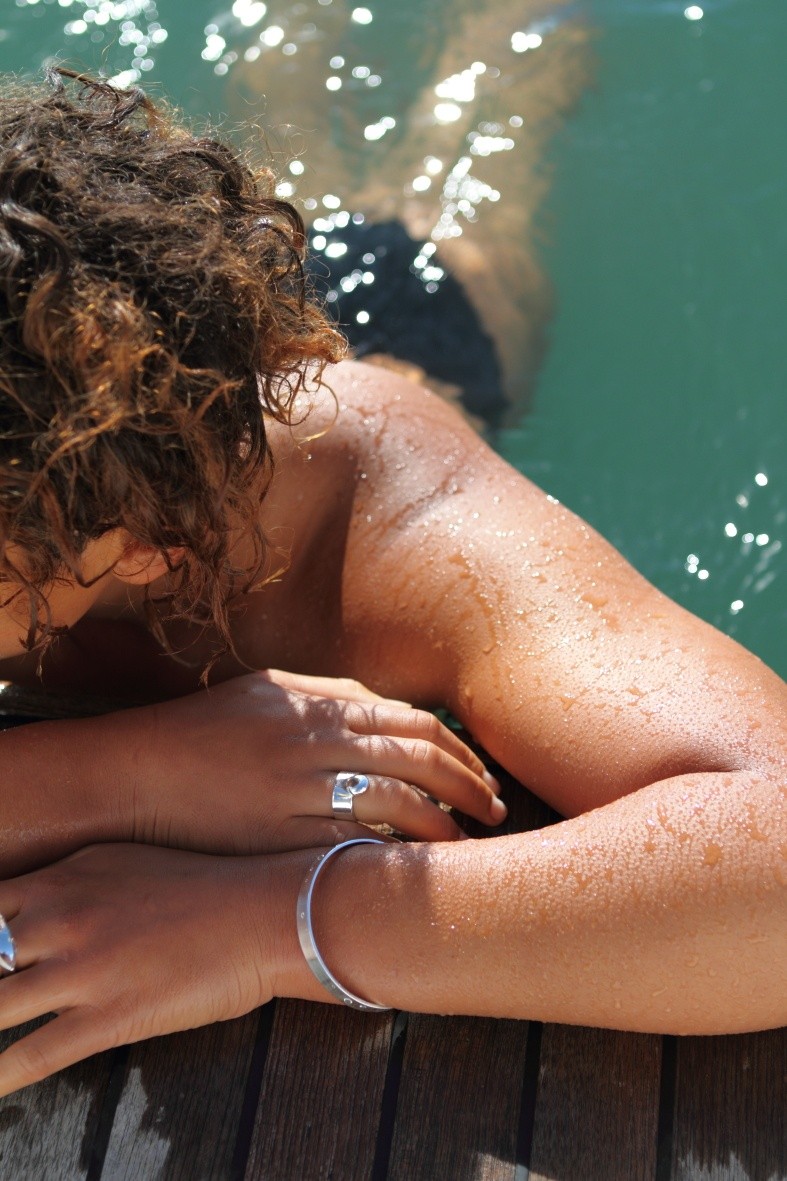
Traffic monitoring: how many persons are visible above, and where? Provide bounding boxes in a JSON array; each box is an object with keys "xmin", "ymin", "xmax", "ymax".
[{"xmin": 0, "ymin": 70, "xmax": 787, "ymax": 1102}]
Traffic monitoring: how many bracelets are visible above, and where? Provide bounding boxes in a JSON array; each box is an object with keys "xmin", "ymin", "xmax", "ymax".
[{"xmin": 297, "ymin": 840, "xmax": 392, "ymax": 1012}]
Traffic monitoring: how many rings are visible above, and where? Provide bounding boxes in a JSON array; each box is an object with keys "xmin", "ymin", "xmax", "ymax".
[
  {"xmin": 0, "ymin": 915, "xmax": 14, "ymax": 975},
  {"xmin": 332, "ymin": 771, "xmax": 368, "ymax": 820}
]
